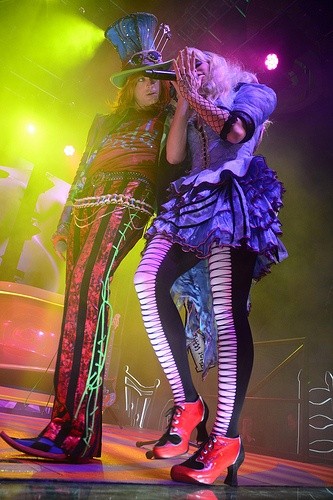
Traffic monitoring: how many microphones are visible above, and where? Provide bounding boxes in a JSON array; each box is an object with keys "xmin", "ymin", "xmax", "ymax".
[{"xmin": 142, "ymin": 69, "xmax": 176, "ymax": 81}]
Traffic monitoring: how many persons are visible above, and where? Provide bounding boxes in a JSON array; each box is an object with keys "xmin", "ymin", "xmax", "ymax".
[
  {"xmin": 0, "ymin": 12, "xmax": 175, "ymax": 463},
  {"xmin": 132, "ymin": 45, "xmax": 289, "ymax": 489}
]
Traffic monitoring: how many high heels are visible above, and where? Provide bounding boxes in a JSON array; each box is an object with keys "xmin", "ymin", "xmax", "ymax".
[
  {"xmin": 152, "ymin": 393, "xmax": 209, "ymax": 459},
  {"xmin": 170, "ymin": 435, "xmax": 245, "ymax": 487}
]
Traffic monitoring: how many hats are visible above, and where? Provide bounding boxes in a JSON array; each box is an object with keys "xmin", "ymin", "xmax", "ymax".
[{"xmin": 104, "ymin": 12, "xmax": 175, "ymax": 89}]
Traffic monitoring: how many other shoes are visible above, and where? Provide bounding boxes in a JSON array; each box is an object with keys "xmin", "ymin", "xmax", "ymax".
[{"xmin": 0, "ymin": 431, "xmax": 93, "ymax": 463}]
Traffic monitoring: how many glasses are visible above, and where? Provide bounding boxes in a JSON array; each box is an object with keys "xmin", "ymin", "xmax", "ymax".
[
  {"xmin": 195, "ymin": 61, "xmax": 202, "ymax": 68},
  {"xmin": 121, "ymin": 50, "xmax": 162, "ymax": 70}
]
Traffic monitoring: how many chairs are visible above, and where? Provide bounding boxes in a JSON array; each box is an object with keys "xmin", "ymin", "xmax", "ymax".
[{"xmin": 121, "ymin": 365, "xmax": 161, "ymax": 428}]
